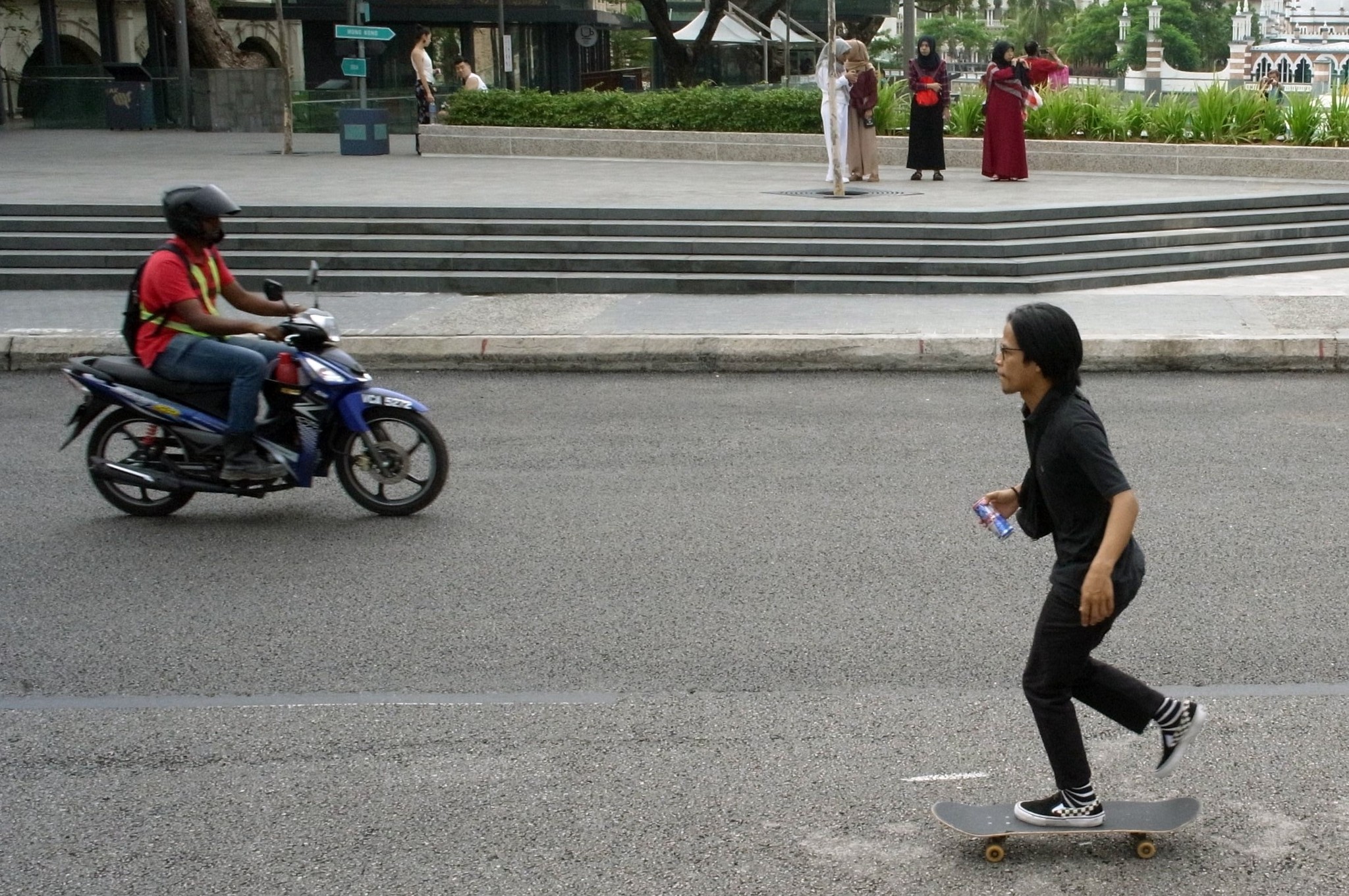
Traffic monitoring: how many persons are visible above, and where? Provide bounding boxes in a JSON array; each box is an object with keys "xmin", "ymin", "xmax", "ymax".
[
  {"xmin": 136, "ymin": 185, "xmax": 307, "ymax": 481},
  {"xmin": 411, "ymin": 24, "xmax": 438, "ymax": 155},
  {"xmin": 815, "ymin": 37, "xmax": 858, "ymax": 183},
  {"xmin": 981, "ymin": 40, "xmax": 1030, "ymax": 179},
  {"xmin": 843, "ymin": 39, "xmax": 879, "ymax": 181},
  {"xmin": 1021, "ymin": 40, "xmax": 1065, "ymax": 93},
  {"xmin": 905, "ymin": 36, "xmax": 946, "ymax": 180},
  {"xmin": 455, "ymin": 57, "xmax": 488, "ymax": 93},
  {"xmin": 978, "ymin": 301, "xmax": 1204, "ymax": 828},
  {"xmin": 1260, "ymin": 70, "xmax": 1284, "ymax": 105}
]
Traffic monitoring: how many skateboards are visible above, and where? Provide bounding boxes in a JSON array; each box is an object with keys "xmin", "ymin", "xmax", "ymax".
[{"xmin": 932, "ymin": 797, "xmax": 1205, "ymax": 862}]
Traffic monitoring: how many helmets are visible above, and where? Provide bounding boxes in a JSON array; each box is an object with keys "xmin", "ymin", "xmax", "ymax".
[{"xmin": 161, "ymin": 186, "xmax": 225, "ymax": 248}]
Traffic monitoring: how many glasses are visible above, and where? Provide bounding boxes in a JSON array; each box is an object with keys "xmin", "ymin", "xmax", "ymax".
[{"xmin": 996, "ymin": 345, "xmax": 1024, "ymax": 360}]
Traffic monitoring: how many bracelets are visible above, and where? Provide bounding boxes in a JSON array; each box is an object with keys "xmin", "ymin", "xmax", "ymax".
[{"xmin": 1011, "ymin": 487, "xmax": 1020, "ymax": 504}]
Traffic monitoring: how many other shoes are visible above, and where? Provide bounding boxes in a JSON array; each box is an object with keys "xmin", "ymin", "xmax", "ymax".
[
  {"xmin": 867, "ymin": 174, "xmax": 879, "ymax": 182},
  {"xmin": 849, "ymin": 173, "xmax": 863, "ymax": 181},
  {"xmin": 841, "ymin": 177, "xmax": 850, "ymax": 183}
]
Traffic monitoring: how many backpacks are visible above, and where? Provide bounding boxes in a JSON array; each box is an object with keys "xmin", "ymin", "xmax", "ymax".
[
  {"xmin": 121, "ymin": 243, "xmax": 192, "ymax": 357},
  {"xmin": 911, "ymin": 58, "xmax": 944, "ymax": 106}
]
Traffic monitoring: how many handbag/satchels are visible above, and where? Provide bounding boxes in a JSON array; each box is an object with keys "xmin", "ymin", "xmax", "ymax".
[
  {"xmin": 981, "ymin": 102, "xmax": 987, "ymax": 117},
  {"xmin": 1015, "ymin": 466, "xmax": 1052, "ymax": 539},
  {"xmin": 865, "ymin": 117, "xmax": 875, "ymax": 127}
]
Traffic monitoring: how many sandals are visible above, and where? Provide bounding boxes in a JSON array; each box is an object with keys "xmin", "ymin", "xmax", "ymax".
[
  {"xmin": 933, "ymin": 172, "xmax": 943, "ymax": 180},
  {"xmin": 1009, "ymin": 177, "xmax": 1018, "ymax": 181},
  {"xmin": 911, "ymin": 172, "xmax": 922, "ymax": 180},
  {"xmin": 991, "ymin": 176, "xmax": 1000, "ymax": 182}
]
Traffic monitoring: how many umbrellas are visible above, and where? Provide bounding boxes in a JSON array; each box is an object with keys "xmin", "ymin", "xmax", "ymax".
[{"xmin": 641, "ymin": 1, "xmax": 828, "ymax": 83}]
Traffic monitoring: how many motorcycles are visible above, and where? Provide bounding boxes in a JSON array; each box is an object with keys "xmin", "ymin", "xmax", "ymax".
[{"xmin": 58, "ymin": 259, "xmax": 450, "ymax": 518}]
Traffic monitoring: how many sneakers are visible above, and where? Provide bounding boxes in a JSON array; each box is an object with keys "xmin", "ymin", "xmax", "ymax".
[
  {"xmin": 1014, "ymin": 791, "xmax": 1106, "ymax": 828},
  {"xmin": 1151, "ymin": 699, "xmax": 1206, "ymax": 779},
  {"xmin": 219, "ymin": 451, "xmax": 288, "ymax": 479}
]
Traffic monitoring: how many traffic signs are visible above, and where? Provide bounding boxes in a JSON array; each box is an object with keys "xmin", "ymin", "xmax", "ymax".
[
  {"xmin": 341, "ymin": 58, "xmax": 367, "ymax": 77},
  {"xmin": 336, "ymin": 25, "xmax": 396, "ymax": 40}
]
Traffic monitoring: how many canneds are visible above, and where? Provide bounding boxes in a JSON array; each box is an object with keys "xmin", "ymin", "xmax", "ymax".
[{"xmin": 972, "ymin": 497, "xmax": 1014, "ymax": 541}]
